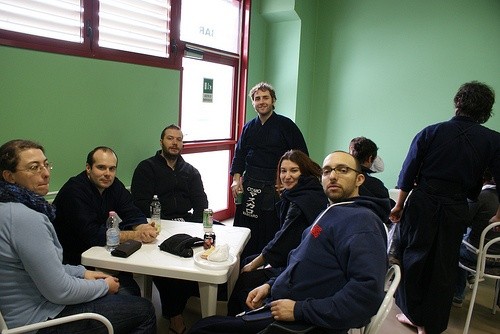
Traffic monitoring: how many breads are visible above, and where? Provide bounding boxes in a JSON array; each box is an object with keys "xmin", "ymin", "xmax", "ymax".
[{"xmin": 201, "ymin": 246, "xmax": 215, "ymax": 259}]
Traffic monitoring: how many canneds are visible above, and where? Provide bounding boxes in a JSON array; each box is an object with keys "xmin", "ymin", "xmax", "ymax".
[
  {"xmin": 203, "ymin": 209, "xmax": 213, "ymax": 228},
  {"xmin": 204, "ymin": 232, "xmax": 215, "ymax": 250}
]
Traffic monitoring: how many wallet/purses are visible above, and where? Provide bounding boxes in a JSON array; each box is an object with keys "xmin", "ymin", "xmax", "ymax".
[{"xmin": 111, "ymin": 239, "xmax": 142, "ymax": 258}]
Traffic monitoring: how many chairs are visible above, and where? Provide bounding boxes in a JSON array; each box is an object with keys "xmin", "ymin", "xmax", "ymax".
[
  {"xmin": 349, "ymin": 265, "xmax": 401, "ymax": 334},
  {"xmin": 459, "ymin": 222, "xmax": 500, "ymax": 334}
]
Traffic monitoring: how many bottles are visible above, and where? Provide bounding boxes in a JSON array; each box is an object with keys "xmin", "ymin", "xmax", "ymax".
[
  {"xmin": 106, "ymin": 211, "xmax": 120, "ymax": 252},
  {"xmin": 150, "ymin": 194, "xmax": 161, "ymax": 233}
]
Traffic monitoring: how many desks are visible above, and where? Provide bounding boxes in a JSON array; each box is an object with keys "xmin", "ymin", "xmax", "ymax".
[{"xmin": 81, "ymin": 218, "xmax": 251, "ymax": 318}]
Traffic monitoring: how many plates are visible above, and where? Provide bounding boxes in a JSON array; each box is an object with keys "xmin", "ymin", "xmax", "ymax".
[{"xmin": 193, "ymin": 251, "xmax": 237, "ymax": 267}]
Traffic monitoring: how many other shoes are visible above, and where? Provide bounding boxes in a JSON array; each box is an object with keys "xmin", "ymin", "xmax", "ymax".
[
  {"xmin": 453, "ymin": 294, "xmax": 462, "ymax": 303},
  {"xmin": 468, "ymin": 272, "xmax": 485, "ymax": 283},
  {"xmin": 169, "ymin": 314, "xmax": 187, "ymax": 334}
]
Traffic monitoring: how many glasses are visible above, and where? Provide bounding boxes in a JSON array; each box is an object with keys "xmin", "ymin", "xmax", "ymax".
[
  {"xmin": 318, "ymin": 165, "xmax": 362, "ymax": 175},
  {"xmin": 17, "ymin": 161, "xmax": 54, "ymax": 173}
]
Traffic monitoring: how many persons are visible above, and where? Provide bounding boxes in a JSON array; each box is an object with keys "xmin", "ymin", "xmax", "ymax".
[
  {"xmin": 347, "ymin": 137, "xmax": 402, "ymax": 263},
  {"xmin": 452, "ymin": 172, "xmax": 500, "ymax": 308},
  {"xmin": 229, "ymin": 83, "xmax": 310, "ymax": 263},
  {"xmin": 189, "ymin": 151, "xmax": 391, "ymax": 334},
  {"xmin": 228, "ymin": 149, "xmax": 330, "ymax": 317},
  {"xmin": 389, "ymin": 81, "xmax": 500, "ymax": 334},
  {"xmin": 131, "ymin": 124, "xmax": 227, "ymax": 334},
  {"xmin": 52, "ymin": 147, "xmax": 158, "ymax": 299},
  {"xmin": 0, "ymin": 139, "xmax": 156, "ymax": 334}
]
